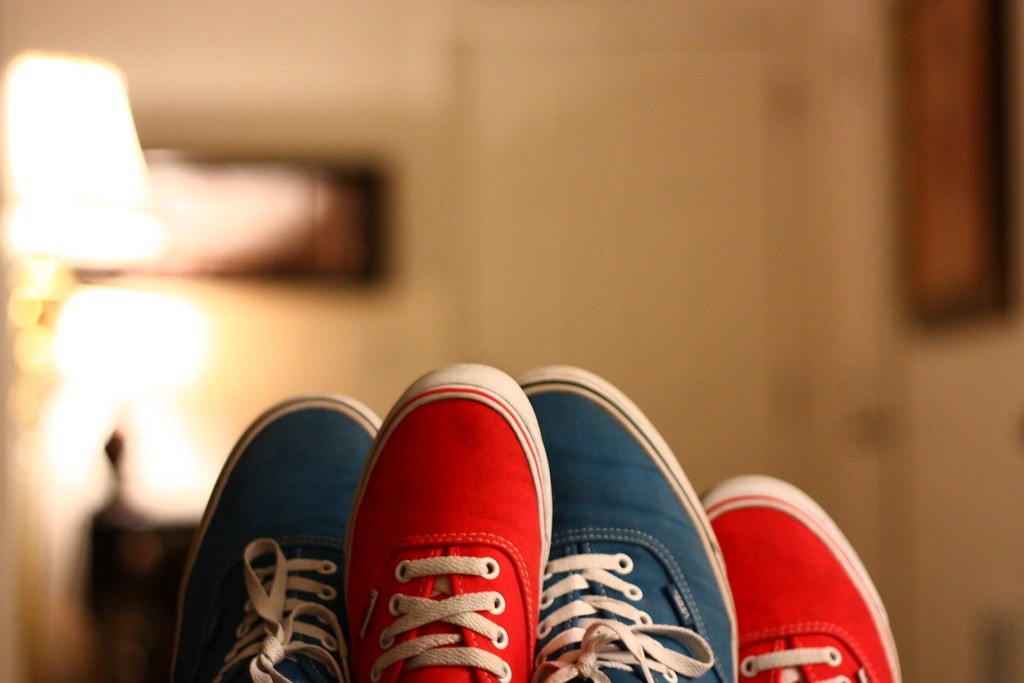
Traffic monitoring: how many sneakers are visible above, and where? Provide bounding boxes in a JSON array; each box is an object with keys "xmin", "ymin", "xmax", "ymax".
[
  {"xmin": 513, "ymin": 366, "xmax": 738, "ymax": 683},
  {"xmin": 170, "ymin": 394, "xmax": 382, "ymax": 683},
  {"xmin": 344, "ymin": 361, "xmax": 553, "ymax": 683},
  {"xmin": 700, "ymin": 472, "xmax": 901, "ymax": 683}
]
